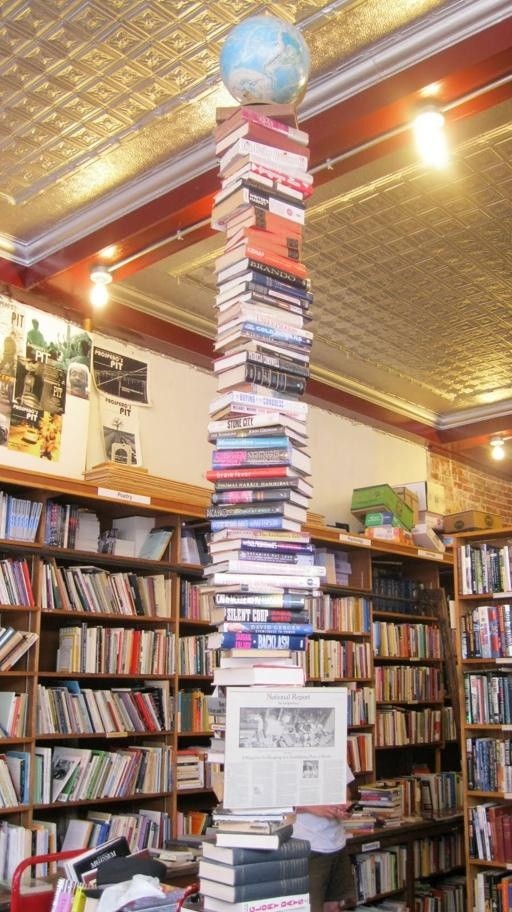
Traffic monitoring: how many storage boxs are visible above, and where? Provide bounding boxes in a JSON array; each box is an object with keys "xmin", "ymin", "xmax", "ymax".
[
  {"xmin": 393, "ymin": 487, "xmax": 419, "ymax": 528},
  {"xmin": 443, "ymin": 510, "xmax": 504, "ymax": 534},
  {"xmin": 350, "ymin": 484, "xmax": 413, "ymax": 532},
  {"xmin": 418, "ymin": 510, "xmax": 445, "ymax": 532}
]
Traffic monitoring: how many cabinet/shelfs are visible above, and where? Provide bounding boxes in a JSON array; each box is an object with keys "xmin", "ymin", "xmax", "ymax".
[
  {"xmin": 0, "ymin": 463, "xmax": 469, "ymax": 912},
  {"xmin": 453, "ymin": 527, "xmax": 512, "ymax": 911}
]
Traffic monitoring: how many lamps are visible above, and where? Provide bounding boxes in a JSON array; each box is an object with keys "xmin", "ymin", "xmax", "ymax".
[
  {"xmin": 404, "ymin": 97, "xmax": 446, "ymax": 141},
  {"xmin": 87, "ymin": 264, "xmax": 113, "ymax": 310},
  {"xmin": 488, "ymin": 434, "xmax": 505, "ymax": 461}
]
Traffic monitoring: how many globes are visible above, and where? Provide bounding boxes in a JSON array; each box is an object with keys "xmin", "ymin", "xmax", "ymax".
[{"xmin": 219, "ymin": 15, "xmax": 310, "ymax": 108}]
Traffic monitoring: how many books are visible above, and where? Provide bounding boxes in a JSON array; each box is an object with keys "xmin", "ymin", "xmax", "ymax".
[
  {"xmin": 2, "ymin": 809, "xmax": 212, "ymax": 910},
  {"xmin": 373, "ymin": 556, "xmax": 440, "ymax": 615},
  {"xmin": 347, "ymin": 730, "xmax": 373, "ymax": 772},
  {"xmin": 1, "ymin": 554, "xmax": 198, "ymax": 620},
  {"xmin": 353, "ymin": 767, "xmax": 468, "ymax": 912},
  {"xmin": 2, "ymin": 623, "xmax": 221, "ymax": 673},
  {"xmin": 371, "ymin": 623, "xmax": 459, "ymax": 748},
  {"xmin": 0, "ymin": 489, "xmax": 209, "ymax": 567},
  {"xmin": 333, "ymin": 684, "xmax": 376, "ymax": 727},
  {"xmin": 1, "ymin": 680, "xmax": 210, "ymax": 737},
  {"xmin": 1, "ymin": 740, "xmax": 221, "ymax": 809},
  {"xmin": 199, "ymin": 105, "xmax": 350, "ymax": 911},
  {"xmin": 458, "ymin": 540, "xmax": 512, "ymax": 912},
  {"xmin": 306, "ymin": 591, "xmax": 372, "ymax": 682}
]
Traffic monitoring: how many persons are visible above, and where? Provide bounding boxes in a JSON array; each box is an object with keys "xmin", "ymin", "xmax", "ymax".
[
  {"xmin": 2, "ymin": 331, "xmax": 17, "ymax": 374},
  {"xmin": 68, "ymin": 366, "xmax": 89, "ymax": 398},
  {"xmin": 290, "ymin": 765, "xmax": 356, "ymax": 909}
]
